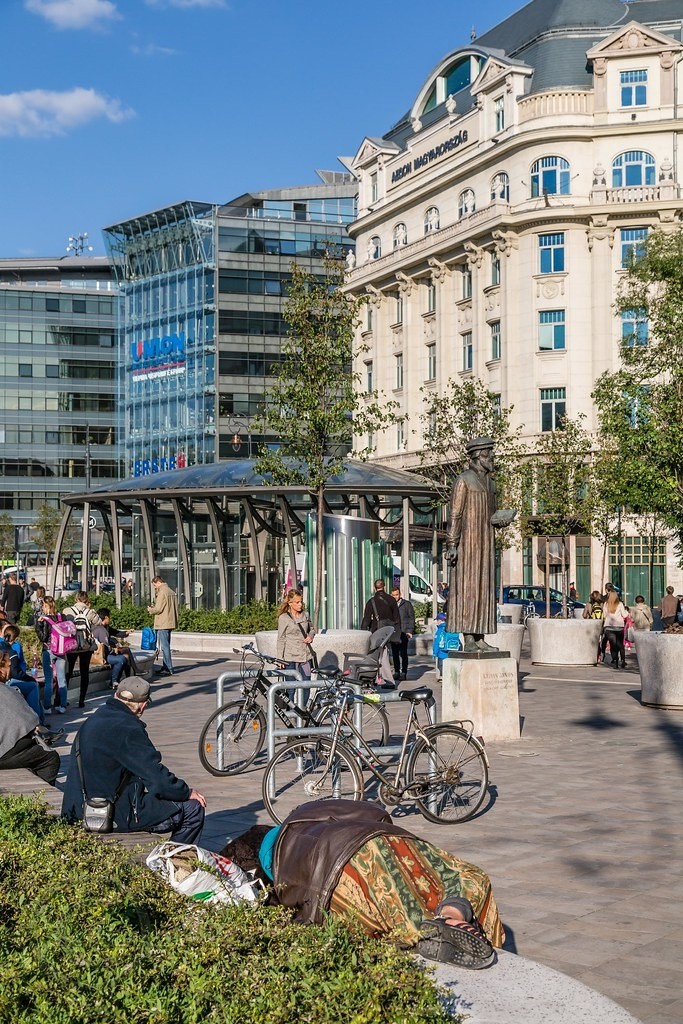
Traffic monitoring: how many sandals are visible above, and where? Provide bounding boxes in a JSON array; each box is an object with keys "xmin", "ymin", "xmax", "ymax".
[
  {"xmin": 419, "ymin": 913, "xmax": 492, "ymax": 959},
  {"xmin": 417, "ymin": 938, "xmax": 496, "ymax": 969}
]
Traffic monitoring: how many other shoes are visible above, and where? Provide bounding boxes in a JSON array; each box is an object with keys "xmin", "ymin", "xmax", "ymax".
[
  {"xmin": 598, "ymin": 655, "xmax": 627, "ymax": 669},
  {"xmin": 41, "ymin": 707, "xmax": 51, "ymax": 715},
  {"xmin": 41, "ymin": 732, "xmax": 64, "ymax": 745},
  {"xmin": 155, "ymin": 667, "xmax": 170, "ymax": 675},
  {"xmin": 43, "ymin": 724, "xmax": 51, "ymax": 729},
  {"xmin": 399, "ymin": 673, "xmax": 406, "ymax": 681},
  {"xmin": 56, "ymin": 728, "xmax": 64, "ymax": 733},
  {"xmin": 134, "ymin": 670, "xmax": 149, "ymax": 676},
  {"xmin": 54, "ymin": 705, "xmax": 66, "ymax": 714},
  {"xmin": 392, "ymin": 671, "xmax": 400, "ymax": 680},
  {"xmin": 110, "ymin": 681, "xmax": 119, "ymax": 688}
]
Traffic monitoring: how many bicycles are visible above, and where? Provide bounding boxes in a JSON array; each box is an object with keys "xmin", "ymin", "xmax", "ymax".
[
  {"xmin": 553, "ymin": 599, "xmax": 575, "ymax": 619},
  {"xmin": 518, "ymin": 601, "xmax": 542, "ymax": 630},
  {"xmin": 260, "ymin": 668, "xmax": 491, "ymax": 827},
  {"xmin": 198, "ymin": 641, "xmax": 391, "ymax": 777}
]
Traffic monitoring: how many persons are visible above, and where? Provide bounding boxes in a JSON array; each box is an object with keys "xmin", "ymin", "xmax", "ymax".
[
  {"xmin": 360, "ymin": 579, "xmax": 401, "ymax": 689},
  {"xmin": 146, "ymin": 576, "xmax": 180, "ymax": 675},
  {"xmin": 276, "ymin": 589, "xmax": 316, "ymax": 725},
  {"xmin": 431, "ymin": 580, "xmax": 460, "ymax": 683},
  {"xmin": 253, "ymin": 798, "xmax": 506, "ymax": 970},
  {"xmin": 60, "ymin": 675, "xmax": 207, "ymax": 845},
  {"xmin": 569, "ymin": 582, "xmax": 576, "ymax": 601},
  {"xmin": 583, "ymin": 583, "xmax": 654, "ymax": 668},
  {"xmin": 444, "ymin": 436, "xmax": 512, "ymax": 652},
  {"xmin": 0, "ymin": 650, "xmax": 61, "ymax": 787},
  {"xmin": 0, "ymin": 572, "xmax": 149, "ymax": 746},
  {"xmin": 657, "ymin": 585, "xmax": 682, "ymax": 630},
  {"xmin": 390, "ymin": 587, "xmax": 415, "ymax": 681}
]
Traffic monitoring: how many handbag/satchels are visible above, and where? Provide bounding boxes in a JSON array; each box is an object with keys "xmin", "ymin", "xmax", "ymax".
[
  {"xmin": 146, "ymin": 841, "xmax": 268, "ymax": 908},
  {"xmin": 141, "ymin": 626, "xmax": 157, "ymax": 650},
  {"xmin": 90, "ymin": 642, "xmax": 105, "ymax": 666},
  {"xmin": 308, "ymin": 653, "xmax": 318, "ymax": 670},
  {"xmin": 377, "ymin": 618, "xmax": 401, "ymax": 642},
  {"xmin": 83, "ymin": 796, "xmax": 114, "ymax": 832}
]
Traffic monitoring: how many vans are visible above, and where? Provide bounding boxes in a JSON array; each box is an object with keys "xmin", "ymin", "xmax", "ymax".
[{"xmin": 283, "ymin": 551, "xmax": 447, "ymax": 608}]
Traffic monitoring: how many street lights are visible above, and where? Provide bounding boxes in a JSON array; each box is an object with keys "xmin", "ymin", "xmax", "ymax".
[
  {"xmin": 82, "ymin": 420, "xmax": 94, "ymax": 584},
  {"xmin": 227, "ymin": 410, "xmax": 271, "ymax": 458}
]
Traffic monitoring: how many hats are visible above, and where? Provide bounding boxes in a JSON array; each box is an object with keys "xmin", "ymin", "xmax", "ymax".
[
  {"xmin": 433, "ymin": 613, "xmax": 446, "ymax": 620},
  {"xmin": 115, "ymin": 675, "xmax": 153, "ymax": 703}
]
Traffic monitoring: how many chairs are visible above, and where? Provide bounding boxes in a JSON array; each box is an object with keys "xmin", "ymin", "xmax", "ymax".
[
  {"xmin": 527, "ymin": 594, "xmax": 534, "ymax": 600},
  {"xmin": 510, "ymin": 592, "xmax": 514, "ymax": 599}
]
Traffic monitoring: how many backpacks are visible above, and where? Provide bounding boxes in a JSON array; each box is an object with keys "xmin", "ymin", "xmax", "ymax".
[
  {"xmin": 41, "ymin": 613, "xmax": 78, "ymax": 656},
  {"xmin": 69, "ymin": 606, "xmax": 95, "ymax": 651},
  {"xmin": 439, "ymin": 630, "xmax": 460, "ymax": 652},
  {"xmin": 589, "ymin": 600, "xmax": 604, "ymax": 624}
]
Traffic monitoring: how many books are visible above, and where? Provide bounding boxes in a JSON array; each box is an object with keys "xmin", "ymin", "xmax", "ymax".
[{"xmin": 491, "ymin": 509, "xmax": 517, "ymax": 525}]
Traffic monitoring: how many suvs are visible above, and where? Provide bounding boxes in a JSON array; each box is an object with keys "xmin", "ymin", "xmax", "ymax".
[{"xmin": 495, "ymin": 584, "xmax": 587, "ymax": 619}]
